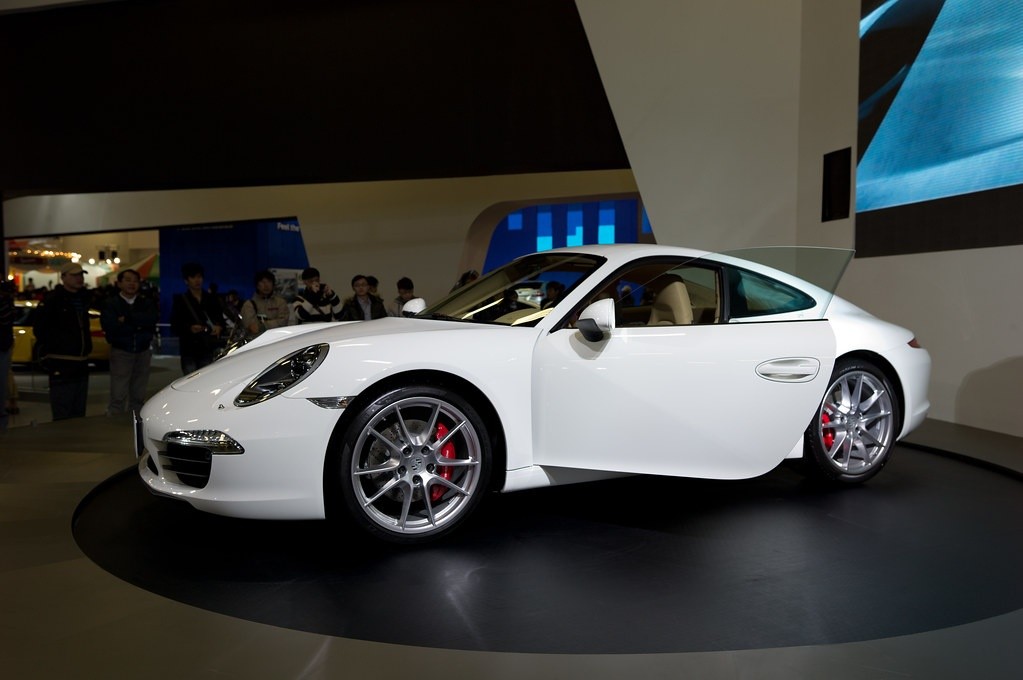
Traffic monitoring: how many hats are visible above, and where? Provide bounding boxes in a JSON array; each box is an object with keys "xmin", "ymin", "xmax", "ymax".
[{"xmin": 60, "ymin": 263, "xmax": 88, "ymax": 275}]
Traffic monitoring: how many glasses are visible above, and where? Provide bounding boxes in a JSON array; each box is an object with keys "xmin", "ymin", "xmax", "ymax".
[{"xmin": 354, "ymin": 283, "xmax": 368, "ymax": 287}]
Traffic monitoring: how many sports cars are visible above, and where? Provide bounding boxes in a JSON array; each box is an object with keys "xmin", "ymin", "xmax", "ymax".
[{"xmin": 131, "ymin": 243, "xmax": 933, "ymax": 552}]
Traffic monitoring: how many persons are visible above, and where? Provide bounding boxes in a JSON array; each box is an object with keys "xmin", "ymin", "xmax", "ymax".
[
  {"xmin": 539, "ymin": 280, "xmax": 565, "ymax": 309},
  {"xmin": 340, "ymin": 275, "xmax": 388, "ymax": 321},
  {"xmin": 31, "ymin": 264, "xmax": 106, "ymax": 421},
  {"xmin": 100, "ymin": 271, "xmax": 158, "ymax": 416},
  {"xmin": 388, "ymin": 277, "xmax": 427, "ymax": 316},
  {"xmin": 0, "ymin": 273, "xmax": 25, "ymax": 414},
  {"xmin": 28, "ymin": 278, "xmax": 35, "ymax": 292},
  {"xmin": 240, "ymin": 271, "xmax": 290, "ymax": 339},
  {"xmin": 208, "ymin": 282, "xmax": 223, "ymax": 297},
  {"xmin": 571, "ymin": 284, "xmax": 634, "ymax": 326},
  {"xmin": 169, "ymin": 260, "xmax": 233, "ymax": 374},
  {"xmin": 493, "ymin": 288, "xmax": 536, "ymax": 320},
  {"xmin": 450, "ymin": 268, "xmax": 479, "ymax": 292},
  {"xmin": 293, "ymin": 267, "xmax": 344, "ymax": 323}
]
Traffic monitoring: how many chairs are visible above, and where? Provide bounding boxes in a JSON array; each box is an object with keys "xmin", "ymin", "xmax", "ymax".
[
  {"xmin": 647, "ymin": 273, "xmax": 695, "ymax": 329},
  {"xmin": 578, "ymin": 289, "xmax": 612, "ymax": 318}
]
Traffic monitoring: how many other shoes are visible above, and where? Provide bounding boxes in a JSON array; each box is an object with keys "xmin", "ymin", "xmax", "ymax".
[{"xmin": 7, "ymin": 407, "xmax": 20, "ymax": 414}]
